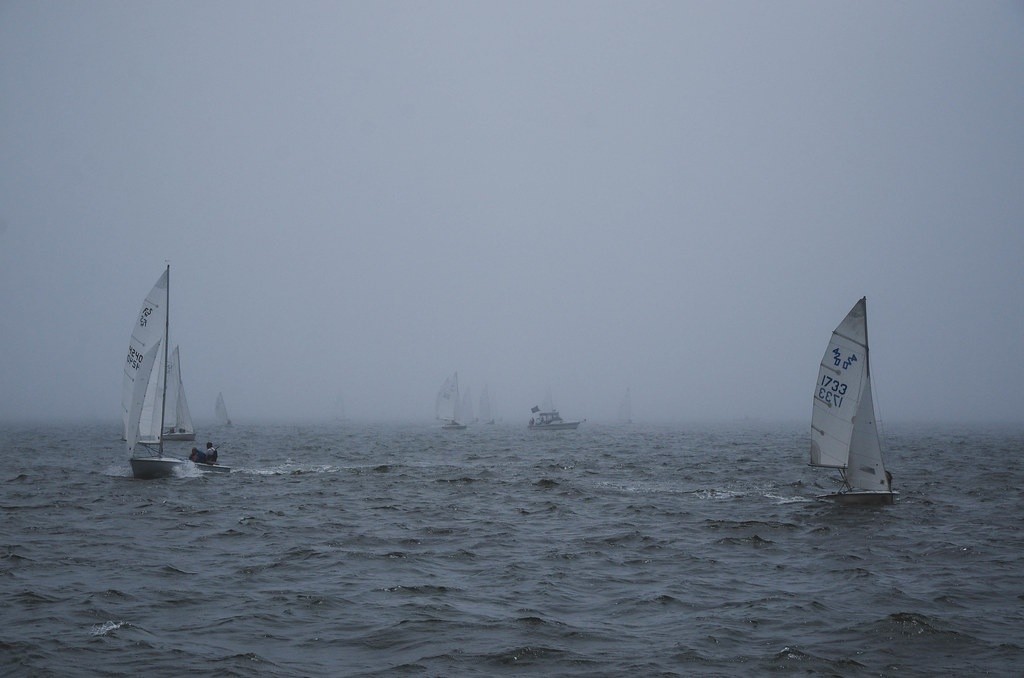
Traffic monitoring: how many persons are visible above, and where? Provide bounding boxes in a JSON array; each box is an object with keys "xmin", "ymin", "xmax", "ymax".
[
  {"xmin": 206, "ymin": 442, "xmax": 217, "ymax": 462},
  {"xmin": 189, "ymin": 448, "xmax": 206, "ymax": 462}
]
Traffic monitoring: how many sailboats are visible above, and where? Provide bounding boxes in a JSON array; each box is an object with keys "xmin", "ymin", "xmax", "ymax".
[
  {"xmin": 214, "ymin": 391, "xmax": 233, "ymax": 427},
  {"xmin": 434, "ymin": 368, "xmax": 466, "ymax": 428},
  {"xmin": 119, "ymin": 265, "xmax": 231, "ymax": 478},
  {"xmin": 476, "ymin": 378, "xmax": 494, "ymax": 424},
  {"xmin": 162, "ymin": 346, "xmax": 197, "ymax": 440},
  {"xmin": 804, "ymin": 293, "xmax": 900, "ymax": 503}
]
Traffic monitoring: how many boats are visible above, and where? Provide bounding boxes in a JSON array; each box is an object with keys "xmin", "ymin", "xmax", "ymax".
[{"xmin": 526, "ymin": 406, "xmax": 580, "ymax": 430}]
